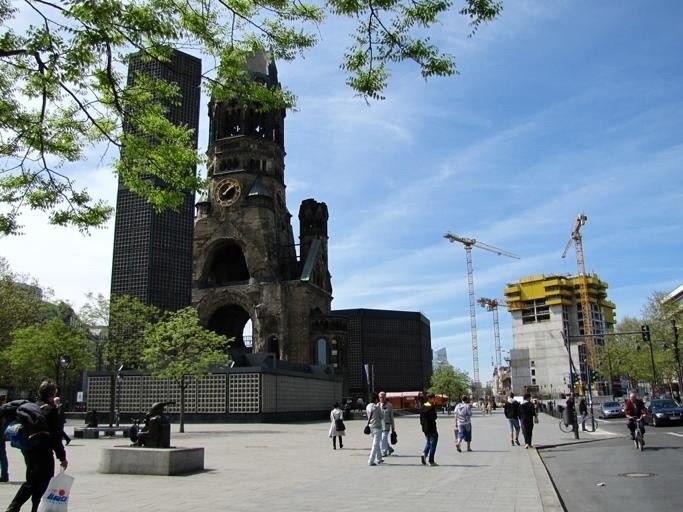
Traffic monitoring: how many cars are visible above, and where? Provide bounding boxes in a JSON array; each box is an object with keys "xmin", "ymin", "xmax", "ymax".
[
  {"xmin": 451, "ymin": 399, "xmax": 506, "ymax": 408},
  {"xmin": 598, "ymin": 402, "xmax": 624, "ymax": 419},
  {"xmin": 642, "ymin": 400, "xmax": 683, "ymax": 426}
]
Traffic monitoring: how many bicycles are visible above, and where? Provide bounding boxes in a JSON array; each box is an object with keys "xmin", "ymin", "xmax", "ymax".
[
  {"xmin": 630, "ymin": 414, "xmax": 647, "ymax": 451},
  {"xmin": 558, "ymin": 413, "xmax": 598, "ymax": 433}
]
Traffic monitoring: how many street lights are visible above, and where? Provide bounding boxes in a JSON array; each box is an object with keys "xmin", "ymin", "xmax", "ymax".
[
  {"xmin": 114, "ymin": 364, "xmax": 127, "ymax": 426},
  {"xmin": 635, "ymin": 338, "xmax": 670, "ymax": 385},
  {"xmin": 59, "ymin": 354, "xmax": 71, "ymax": 406}
]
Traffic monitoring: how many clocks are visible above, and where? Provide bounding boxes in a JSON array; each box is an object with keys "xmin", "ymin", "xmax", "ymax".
[{"xmin": 213, "ymin": 177, "xmax": 241, "ymax": 207}]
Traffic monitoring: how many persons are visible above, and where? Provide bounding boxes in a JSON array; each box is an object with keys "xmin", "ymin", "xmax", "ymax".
[
  {"xmin": 420, "ymin": 394, "xmax": 438, "ymax": 465},
  {"xmin": 624, "ymin": 393, "xmax": 649, "ymax": 445},
  {"xmin": 579, "ymin": 400, "xmax": 588, "ymax": 432},
  {"xmin": 130, "ymin": 402, "xmax": 170, "ymax": 447},
  {"xmin": 441, "ymin": 395, "xmax": 496, "ymax": 415},
  {"xmin": 562, "ymin": 398, "xmax": 575, "ymax": 431},
  {"xmin": 454, "ymin": 395, "xmax": 472, "ymax": 452},
  {"xmin": 643, "ymin": 392, "xmax": 651, "ymax": 403},
  {"xmin": 0, "ymin": 378, "xmax": 71, "ymax": 512},
  {"xmin": 518, "ymin": 393, "xmax": 538, "ymax": 449},
  {"xmin": 504, "ymin": 392, "xmax": 519, "ymax": 445},
  {"xmin": 329, "ymin": 391, "xmax": 394, "ymax": 465}
]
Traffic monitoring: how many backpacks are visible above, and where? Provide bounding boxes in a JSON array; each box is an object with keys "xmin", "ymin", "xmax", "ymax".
[{"xmin": 2, "ymin": 404, "xmax": 48, "ymax": 449}]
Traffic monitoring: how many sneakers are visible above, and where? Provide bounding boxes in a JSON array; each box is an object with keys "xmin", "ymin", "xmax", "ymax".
[
  {"xmin": 368, "ymin": 449, "xmax": 394, "ymax": 466},
  {"xmin": 421, "ymin": 453, "xmax": 438, "ymax": 466}
]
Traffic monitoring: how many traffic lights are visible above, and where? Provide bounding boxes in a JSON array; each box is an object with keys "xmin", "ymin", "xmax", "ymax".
[
  {"xmin": 574, "ymin": 384, "xmax": 582, "ymax": 395},
  {"xmin": 590, "ymin": 370, "xmax": 598, "ymax": 384},
  {"xmin": 573, "ymin": 373, "xmax": 580, "ymax": 383}
]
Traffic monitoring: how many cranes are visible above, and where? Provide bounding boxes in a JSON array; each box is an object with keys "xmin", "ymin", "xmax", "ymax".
[
  {"xmin": 476, "ymin": 297, "xmax": 514, "ymax": 395},
  {"xmin": 445, "ymin": 231, "xmax": 521, "ymax": 403},
  {"xmin": 561, "ymin": 213, "xmax": 598, "ymax": 391}
]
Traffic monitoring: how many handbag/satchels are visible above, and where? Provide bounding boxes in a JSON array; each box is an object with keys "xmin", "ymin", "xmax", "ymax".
[
  {"xmin": 391, "ymin": 431, "xmax": 397, "ymax": 444},
  {"xmin": 364, "ymin": 426, "xmax": 370, "ymax": 434},
  {"xmin": 37, "ymin": 466, "xmax": 74, "ymax": 512},
  {"xmin": 335, "ymin": 419, "xmax": 345, "ymax": 431}
]
